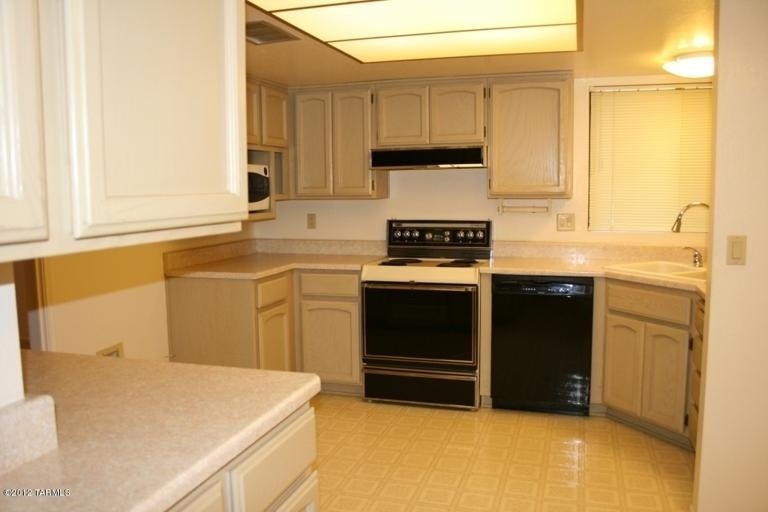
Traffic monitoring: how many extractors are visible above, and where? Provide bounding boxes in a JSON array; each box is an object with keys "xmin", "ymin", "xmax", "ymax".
[{"xmin": 368, "ymin": 146, "xmax": 486, "ymax": 172}]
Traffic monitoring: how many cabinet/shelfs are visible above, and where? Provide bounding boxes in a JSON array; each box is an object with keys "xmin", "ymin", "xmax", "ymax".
[
  {"xmin": 157, "ymin": 391, "xmax": 318, "ymax": 511},
  {"xmin": 287, "ymin": 85, "xmax": 388, "ymax": 199},
  {"xmin": 373, "ymin": 79, "xmax": 486, "ymax": 147},
  {"xmin": 604, "ymin": 272, "xmax": 692, "ymax": 453},
  {"xmin": 247, "ymin": 74, "xmax": 287, "ymax": 223},
  {"xmin": 487, "ymin": 70, "xmax": 573, "ymax": 198},
  {"xmin": 165, "ymin": 264, "xmax": 296, "ymax": 372},
  {"xmin": 294, "ymin": 264, "xmax": 362, "ymax": 396},
  {"xmin": 687, "ymin": 288, "xmax": 706, "ymax": 445},
  {"xmin": 0, "ymin": 0, "xmax": 249, "ymax": 264}
]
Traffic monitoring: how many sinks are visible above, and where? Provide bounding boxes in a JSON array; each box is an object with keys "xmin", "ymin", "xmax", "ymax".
[
  {"xmin": 606, "ymin": 253, "xmax": 695, "ymax": 276},
  {"xmin": 680, "ymin": 263, "xmax": 708, "ymax": 282}
]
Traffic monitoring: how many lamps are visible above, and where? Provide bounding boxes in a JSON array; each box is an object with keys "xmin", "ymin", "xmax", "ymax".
[{"xmin": 661, "ymin": 51, "xmax": 714, "ymax": 81}]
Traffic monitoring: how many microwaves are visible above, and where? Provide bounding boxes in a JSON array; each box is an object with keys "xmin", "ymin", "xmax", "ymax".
[{"xmin": 246, "ymin": 165, "xmax": 270, "ymax": 213}]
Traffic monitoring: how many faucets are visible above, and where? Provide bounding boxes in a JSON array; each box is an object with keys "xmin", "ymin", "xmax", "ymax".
[{"xmin": 673, "ymin": 200, "xmax": 710, "ymax": 234}]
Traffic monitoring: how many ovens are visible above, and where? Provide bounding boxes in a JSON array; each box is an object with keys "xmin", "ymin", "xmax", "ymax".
[{"xmin": 359, "ymin": 266, "xmax": 480, "ymax": 379}]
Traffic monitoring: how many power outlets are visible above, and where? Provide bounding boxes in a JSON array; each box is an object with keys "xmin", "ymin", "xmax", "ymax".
[{"xmin": 307, "ymin": 215, "xmax": 319, "ymax": 231}]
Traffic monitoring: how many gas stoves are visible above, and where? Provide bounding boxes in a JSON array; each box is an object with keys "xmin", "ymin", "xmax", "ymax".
[{"xmin": 377, "ymin": 256, "xmax": 479, "ymax": 269}]
